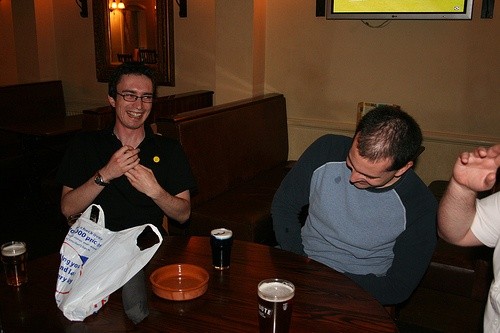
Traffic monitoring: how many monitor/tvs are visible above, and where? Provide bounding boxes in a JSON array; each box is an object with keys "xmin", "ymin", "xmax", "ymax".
[{"xmin": 325, "ymin": 0, "xmax": 474, "ymax": 21}]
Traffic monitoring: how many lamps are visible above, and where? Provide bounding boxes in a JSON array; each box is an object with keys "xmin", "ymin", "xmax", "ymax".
[{"xmin": 112, "ymin": 0, "xmax": 125, "ymax": 9}]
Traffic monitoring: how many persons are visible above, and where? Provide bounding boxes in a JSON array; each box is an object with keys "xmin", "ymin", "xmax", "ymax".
[
  {"xmin": 60, "ymin": 59, "xmax": 198, "ymax": 251},
  {"xmin": 435, "ymin": 141, "xmax": 500, "ymax": 333},
  {"xmin": 271, "ymin": 106, "xmax": 442, "ymax": 316}
]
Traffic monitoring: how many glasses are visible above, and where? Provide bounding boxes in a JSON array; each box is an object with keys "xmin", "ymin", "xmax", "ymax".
[{"xmin": 116, "ymin": 92, "xmax": 153, "ymax": 103}]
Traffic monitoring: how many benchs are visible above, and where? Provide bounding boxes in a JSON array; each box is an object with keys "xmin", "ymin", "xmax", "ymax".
[
  {"xmin": 0, "ymin": 80, "xmax": 306, "ymax": 245},
  {"xmin": 420, "ymin": 169, "xmax": 500, "ymax": 304}
]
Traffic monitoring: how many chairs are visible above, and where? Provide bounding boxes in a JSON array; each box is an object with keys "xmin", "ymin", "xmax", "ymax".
[
  {"xmin": 117, "ymin": 53, "xmax": 134, "ymax": 63},
  {"xmin": 139, "ymin": 47, "xmax": 158, "ymax": 64}
]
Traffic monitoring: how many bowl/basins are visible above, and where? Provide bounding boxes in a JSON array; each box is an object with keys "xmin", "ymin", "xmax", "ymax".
[{"xmin": 149, "ymin": 263, "xmax": 210, "ymax": 301}]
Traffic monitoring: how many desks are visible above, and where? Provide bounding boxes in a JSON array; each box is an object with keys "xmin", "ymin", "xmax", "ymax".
[{"xmin": 0, "ymin": 239, "xmax": 398, "ymax": 333}]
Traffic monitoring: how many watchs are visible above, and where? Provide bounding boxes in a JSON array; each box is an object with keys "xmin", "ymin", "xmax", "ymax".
[{"xmin": 94, "ymin": 171, "xmax": 109, "ymax": 187}]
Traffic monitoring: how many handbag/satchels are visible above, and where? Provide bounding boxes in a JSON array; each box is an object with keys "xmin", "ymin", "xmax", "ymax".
[{"xmin": 55, "ymin": 204, "xmax": 163, "ymax": 323}]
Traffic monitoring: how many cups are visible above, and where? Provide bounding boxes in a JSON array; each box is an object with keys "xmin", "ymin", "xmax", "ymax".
[
  {"xmin": 1, "ymin": 241, "xmax": 31, "ymax": 287},
  {"xmin": 257, "ymin": 278, "xmax": 295, "ymax": 333},
  {"xmin": 207, "ymin": 226, "xmax": 233, "ymax": 271},
  {"xmin": 67, "ymin": 212, "xmax": 97, "ymax": 230}
]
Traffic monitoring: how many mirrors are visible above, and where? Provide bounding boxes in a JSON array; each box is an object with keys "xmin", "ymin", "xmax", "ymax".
[{"xmin": 94, "ymin": 0, "xmax": 175, "ymax": 87}]
